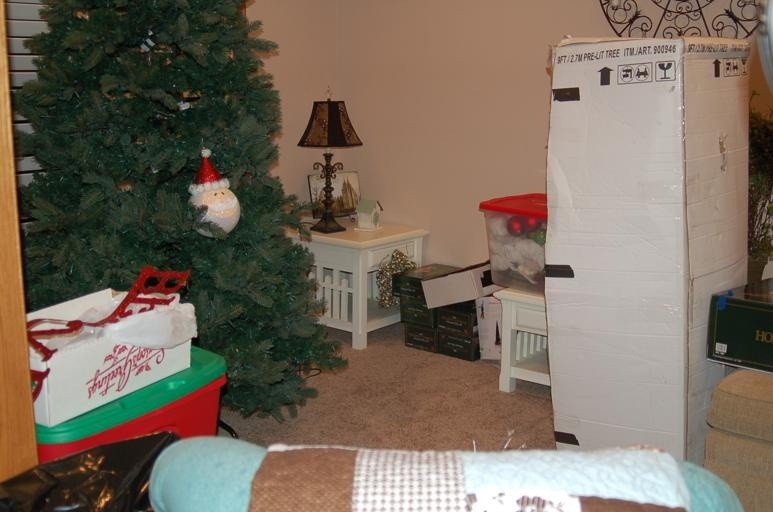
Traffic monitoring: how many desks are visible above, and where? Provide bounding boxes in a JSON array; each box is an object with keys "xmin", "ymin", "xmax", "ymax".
[
  {"xmin": 491, "ymin": 286, "xmax": 551, "ymax": 395},
  {"xmin": 283, "ymin": 214, "xmax": 428, "ymax": 352}
]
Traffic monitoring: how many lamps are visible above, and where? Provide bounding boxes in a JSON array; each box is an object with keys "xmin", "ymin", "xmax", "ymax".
[{"xmin": 294, "ymin": 82, "xmax": 364, "ymax": 235}]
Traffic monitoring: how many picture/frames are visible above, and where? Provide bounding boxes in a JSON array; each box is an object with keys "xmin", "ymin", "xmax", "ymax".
[{"xmin": 306, "ymin": 170, "xmax": 362, "ymax": 219}]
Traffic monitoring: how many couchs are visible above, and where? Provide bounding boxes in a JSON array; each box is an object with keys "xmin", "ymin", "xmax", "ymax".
[
  {"xmin": 144, "ymin": 427, "xmax": 747, "ymax": 511},
  {"xmin": 699, "ymin": 366, "xmax": 771, "ymax": 512}
]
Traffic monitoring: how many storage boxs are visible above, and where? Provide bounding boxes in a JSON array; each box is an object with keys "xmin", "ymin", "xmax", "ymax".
[
  {"xmin": 544, "ymin": 30, "xmax": 751, "ymax": 454},
  {"xmin": 704, "ymin": 279, "xmax": 773, "ymax": 373},
  {"xmin": 391, "ymin": 259, "xmax": 501, "ymax": 362},
  {"xmin": 477, "ymin": 192, "xmax": 545, "ymax": 297},
  {"xmin": 35, "ymin": 343, "xmax": 230, "ymax": 465},
  {"xmin": 21, "ymin": 286, "xmax": 202, "ymax": 428}
]
